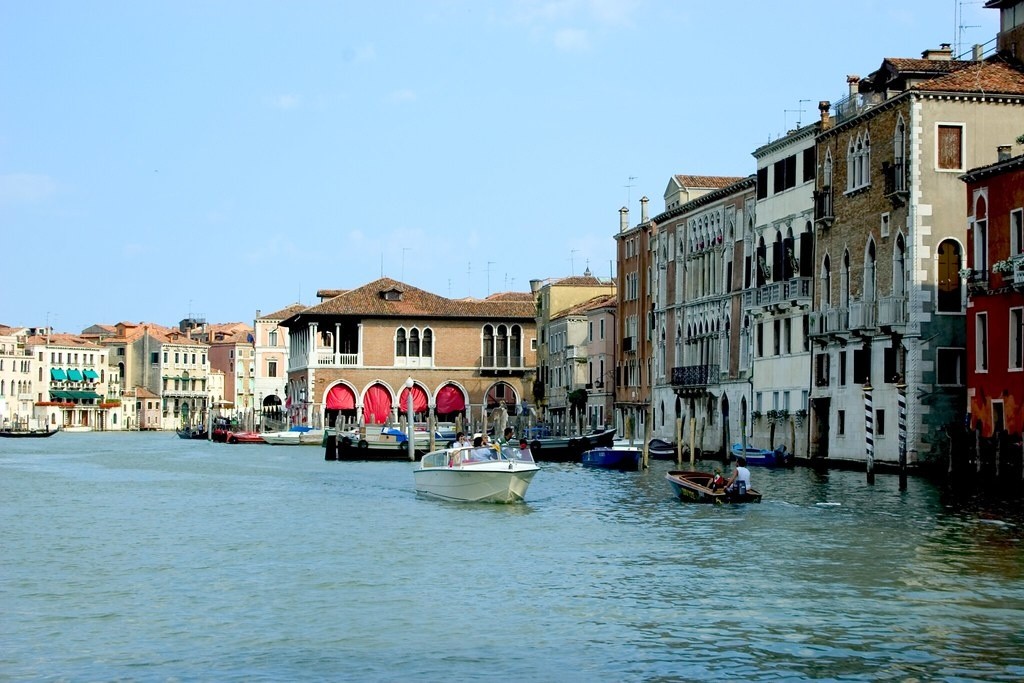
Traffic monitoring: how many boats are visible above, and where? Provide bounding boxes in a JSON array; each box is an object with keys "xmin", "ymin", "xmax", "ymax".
[
  {"xmin": 414, "ymin": 446, "xmax": 541, "ymax": 505},
  {"xmin": 175, "ymin": 427, "xmax": 209, "ymax": 439},
  {"xmin": 580, "ymin": 445, "xmax": 641, "ymax": 473},
  {"xmin": 733, "ymin": 442, "xmax": 787, "ymax": 467},
  {"xmin": 0, "ymin": 427, "xmax": 60, "ymax": 438},
  {"xmin": 649, "ymin": 438, "xmax": 701, "ymax": 462},
  {"xmin": 664, "ymin": 470, "xmax": 762, "ymax": 504},
  {"xmin": 338, "ymin": 422, "xmax": 618, "ymax": 463},
  {"xmin": 230, "ymin": 425, "xmax": 337, "ymax": 445},
  {"xmin": 212, "ymin": 423, "xmax": 234, "ymax": 443}
]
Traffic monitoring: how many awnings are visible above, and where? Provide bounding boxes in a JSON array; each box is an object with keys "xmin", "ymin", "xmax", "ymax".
[
  {"xmin": 51, "ymin": 368, "xmax": 68, "ymax": 380},
  {"xmin": 82, "ymin": 371, "xmax": 99, "ymax": 379},
  {"xmin": 66, "ymin": 370, "xmax": 82, "ymax": 381},
  {"xmin": 49, "ymin": 391, "xmax": 103, "ymax": 400}
]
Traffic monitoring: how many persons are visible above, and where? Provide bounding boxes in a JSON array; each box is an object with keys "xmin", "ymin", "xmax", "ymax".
[
  {"xmin": 521, "ymin": 398, "xmax": 528, "ymax": 416},
  {"xmin": 453, "ymin": 432, "xmax": 472, "ymax": 461},
  {"xmin": 725, "ymin": 458, "xmax": 751, "ymax": 491},
  {"xmin": 489, "ymin": 428, "xmax": 513, "ymax": 460},
  {"xmin": 470, "ymin": 437, "xmax": 493, "ymax": 460},
  {"xmin": 707, "ymin": 467, "xmax": 724, "ymax": 488}
]
